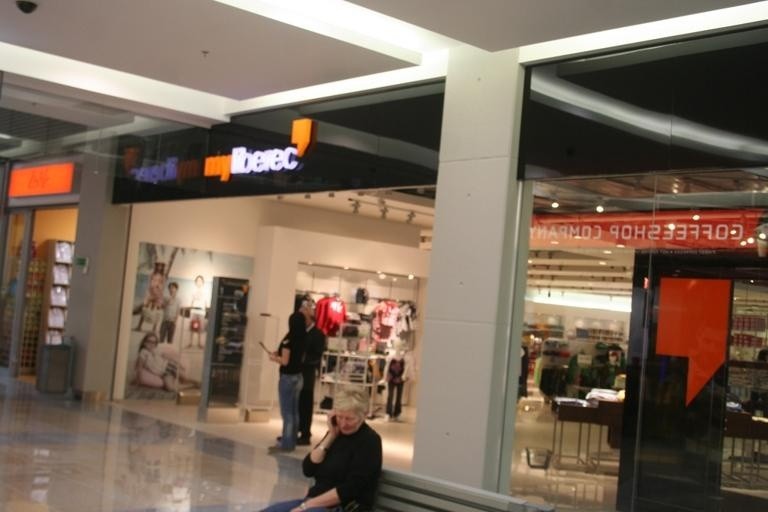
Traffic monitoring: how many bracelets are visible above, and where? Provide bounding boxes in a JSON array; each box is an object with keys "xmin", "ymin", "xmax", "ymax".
[
  {"xmin": 319, "ymin": 443, "xmax": 330, "ymax": 452},
  {"xmin": 299, "ymin": 501, "xmax": 308, "ymax": 510}
]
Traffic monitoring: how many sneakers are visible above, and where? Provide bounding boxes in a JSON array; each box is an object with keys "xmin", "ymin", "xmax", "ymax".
[{"xmin": 269, "ymin": 432, "xmax": 310, "ymax": 452}]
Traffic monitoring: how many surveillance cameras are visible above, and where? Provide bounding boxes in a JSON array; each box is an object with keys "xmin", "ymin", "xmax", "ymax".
[{"xmin": 16, "ymin": 0, "xmax": 37, "ymax": 14}]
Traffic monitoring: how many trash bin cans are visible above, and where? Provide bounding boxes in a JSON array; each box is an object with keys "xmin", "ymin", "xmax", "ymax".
[{"xmin": 36, "ymin": 333, "xmax": 76, "ymax": 394}]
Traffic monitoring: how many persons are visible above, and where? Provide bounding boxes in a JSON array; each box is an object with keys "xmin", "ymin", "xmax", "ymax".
[
  {"xmin": 156, "ymin": 280, "xmax": 182, "ymax": 347},
  {"xmin": 184, "ymin": 275, "xmax": 208, "ymax": 349},
  {"xmin": 267, "ymin": 312, "xmax": 314, "ymax": 452},
  {"xmin": 257, "ymin": 385, "xmax": 383, "ymax": 511},
  {"xmin": 130, "ymin": 334, "xmax": 202, "ymax": 393},
  {"xmin": 381, "ymin": 344, "xmax": 410, "ymax": 424},
  {"xmin": 297, "ymin": 299, "xmax": 328, "ymax": 446},
  {"xmin": 132, "ymin": 261, "xmax": 166, "ymax": 335}
]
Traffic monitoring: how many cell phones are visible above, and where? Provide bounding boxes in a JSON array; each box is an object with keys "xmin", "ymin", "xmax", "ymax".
[{"xmin": 331, "ymin": 416, "xmax": 337, "ymax": 428}]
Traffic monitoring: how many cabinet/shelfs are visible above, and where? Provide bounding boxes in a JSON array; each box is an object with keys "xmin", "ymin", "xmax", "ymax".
[
  {"xmin": 197, "ymin": 277, "xmax": 250, "ymax": 423},
  {"xmin": 315, "ymin": 352, "xmax": 391, "ymax": 419},
  {"xmin": 40, "ymin": 240, "xmax": 74, "ymax": 345}
]
